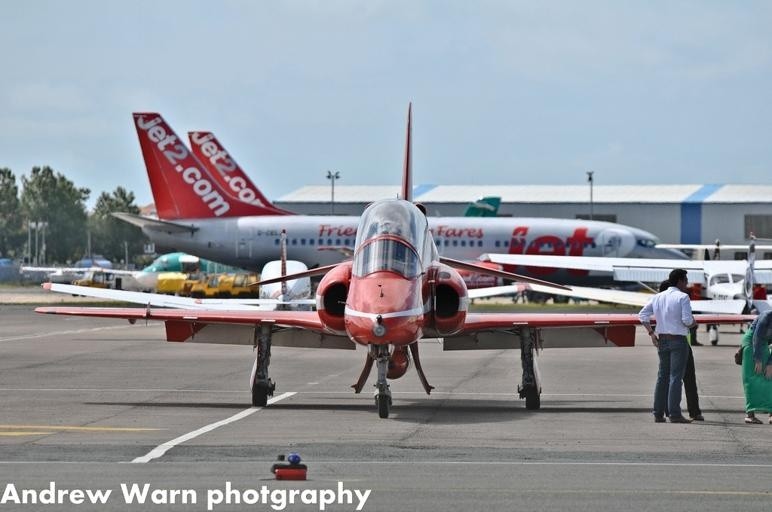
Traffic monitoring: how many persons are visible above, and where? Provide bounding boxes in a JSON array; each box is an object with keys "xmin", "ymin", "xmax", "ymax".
[
  {"xmin": 749, "ymin": 284, "xmax": 769, "ymax": 301},
  {"xmin": 655, "ymin": 278, "xmax": 706, "ymax": 422},
  {"xmin": 638, "ymin": 269, "xmax": 700, "ymax": 423},
  {"xmin": 737, "ymin": 309, "xmax": 772, "ymax": 425},
  {"xmin": 690, "ymin": 322, "xmax": 703, "ymax": 347}
]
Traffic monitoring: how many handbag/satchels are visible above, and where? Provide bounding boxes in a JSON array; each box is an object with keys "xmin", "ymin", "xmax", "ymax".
[{"xmin": 735, "ymin": 346, "xmax": 745, "ymax": 365}]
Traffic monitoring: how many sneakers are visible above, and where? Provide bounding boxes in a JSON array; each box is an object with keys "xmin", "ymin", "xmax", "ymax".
[
  {"xmin": 745, "ymin": 417, "xmax": 762, "ymax": 424},
  {"xmin": 655, "ymin": 415, "xmax": 704, "ymax": 423}
]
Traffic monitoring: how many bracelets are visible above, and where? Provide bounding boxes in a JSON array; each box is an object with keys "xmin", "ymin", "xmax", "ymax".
[{"xmin": 648, "ymin": 330, "xmax": 653, "ymax": 337}]
